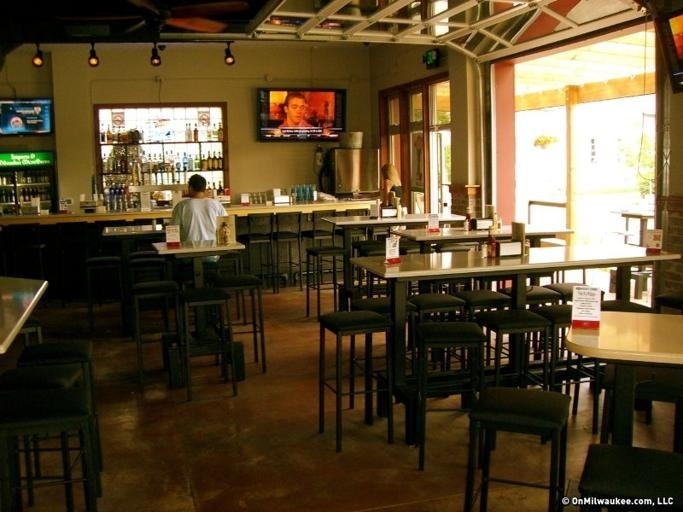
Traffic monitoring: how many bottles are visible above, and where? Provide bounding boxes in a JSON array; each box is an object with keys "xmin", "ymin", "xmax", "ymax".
[
  {"xmin": 174, "ymin": 181, "xmax": 181, "ymax": 194},
  {"xmin": 249, "ymin": 191, "xmax": 268, "ymax": 204},
  {"xmin": 463, "ymin": 207, "xmax": 473, "ymax": 231},
  {"xmin": 206, "ymin": 181, "xmax": 224, "ymax": 199},
  {"xmin": 100, "ymin": 122, "xmax": 224, "ymax": 173},
  {"xmin": 219, "ymin": 221, "xmax": 230, "ymax": 245},
  {"xmin": 0, "ymin": 170, "xmax": 50, "ymax": 214},
  {"xmin": 91, "ymin": 175, "xmax": 98, "ymax": 201},
  {"xmin": 315, "ymin": 143, "xmax": 324, "ymax": 166},
  {"xmin": 281, "ymin": 184, "xmax": 318, "ymax": 203},
  {"xmin": 105, "ymin": 183, "xmax": 139, "ymax": 212},
  {"xmin": 486, "ymin": 226, "xmax": 497, "ymax": 258},
  {"xmin": 525, "ymin": 239, "xmax": 530, "ymax": 256},
  {"xmin": 497, "ymin": 218, "xmax": 502, "ymax": 231}
]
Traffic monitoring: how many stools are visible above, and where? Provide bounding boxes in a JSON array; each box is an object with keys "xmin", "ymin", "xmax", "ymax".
[
  {"xmin": 129, "ymin": 280, "xmax": 184, "ymax": 392},
  {"xmin": 19, "ymin": 317, "xmax": 46, "ymax": 347},
  {"xmin": 185, "ymin": 288, "xmax": 238, "ymax": 400},
  {"xmin": 0, "ymin": 363, "xmax": 102, "ymax": 512},
  {"xmin": 15, "ymin": 338, "xmax": 104, "ymax": 498},
  {"xmin": 215, "ymin": 273, "xmax": 267, "ymax": 385}
]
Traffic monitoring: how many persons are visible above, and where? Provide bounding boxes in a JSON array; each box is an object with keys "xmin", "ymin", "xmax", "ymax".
[
  {"xmin": 376, "ymin": 163, "xmax": 401, "ymax": 241},
  {"xmin": 160, "ymin": 174, "xmax": 228, "ymax": 288},
  {"xmin": 277, "ymin": 92, "xmax": 315, "ymax": 129}
]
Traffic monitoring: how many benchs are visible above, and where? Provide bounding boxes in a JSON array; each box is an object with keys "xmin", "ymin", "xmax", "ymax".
[{"xmin": 621, "ymin": 211, "xmax": 654, "ymax": 248}]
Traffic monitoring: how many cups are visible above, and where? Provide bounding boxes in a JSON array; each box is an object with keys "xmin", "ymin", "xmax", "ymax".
[
  {"xmin": 79, "ymin": 193, "xmax": 85, "ymax": 201},
  {"xmin": 99, "ymin": 193, "xmax": 104, "ymax": 200}
]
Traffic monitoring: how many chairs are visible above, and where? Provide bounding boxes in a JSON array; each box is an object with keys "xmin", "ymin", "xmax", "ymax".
[
  {"xmin": 236, "ymin": 209, "xmax": 370, "ymax": 294},
  {"xmin": 0, "ymin": 218, "xmax": 166, "ymax": 308}
]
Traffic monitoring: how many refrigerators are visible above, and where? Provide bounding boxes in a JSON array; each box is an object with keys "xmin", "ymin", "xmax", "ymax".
[
  {"xmin": 327, "ymin": 147, "xmax": 381, "ymax": 199},
  {"xmin": 0, "ymin": 150, "xmax": 58, "ymax": 217}
]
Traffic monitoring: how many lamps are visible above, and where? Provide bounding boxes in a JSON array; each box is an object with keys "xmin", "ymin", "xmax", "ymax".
[
  {"xmin": 32, "ymin": 43, "xmax": 44, "ymax": 67},
  {"xmin": 88, "ymin": 42, "xmax": 99, "ymax": 67},
  {"xmin": 151, "ymin": 41, "xmax": 161, "ymax": 66},
  {"xmin": 224, "ymin": 41, "xmax": 234, "ymax": 64}
]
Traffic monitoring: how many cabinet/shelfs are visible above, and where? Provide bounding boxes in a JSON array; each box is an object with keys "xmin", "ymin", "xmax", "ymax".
[{"xmin": 93, "ymin": 101, "xmax": 230, "ymax": 211}]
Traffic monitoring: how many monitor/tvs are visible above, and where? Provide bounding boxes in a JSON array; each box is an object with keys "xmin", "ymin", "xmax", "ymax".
[
  {"xmin": 0, "ymin": 98, "xmax": 53, "ymax": 136},
  {"xmin": 256, "ymin": 87, "xmax": 347, "ymax": 143}
]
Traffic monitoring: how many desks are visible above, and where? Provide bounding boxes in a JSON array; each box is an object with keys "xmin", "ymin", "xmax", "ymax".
[{"xmin": 152, "ymin": 239, "xmax": 247, "ymax": 357}]
